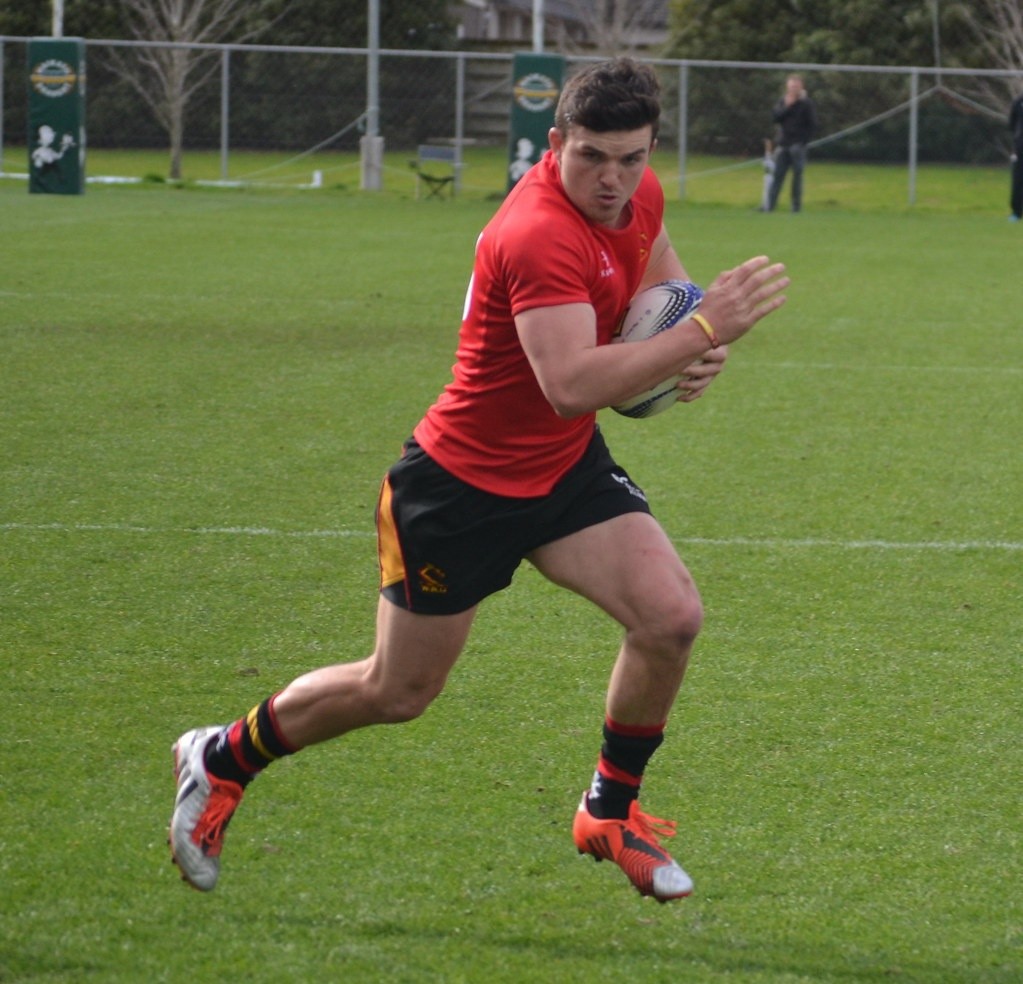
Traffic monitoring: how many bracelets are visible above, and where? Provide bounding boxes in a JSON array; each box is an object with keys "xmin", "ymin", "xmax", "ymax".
[{"xmin": 690, "ymin": 313, "xmax": 721, "ymax": 350}]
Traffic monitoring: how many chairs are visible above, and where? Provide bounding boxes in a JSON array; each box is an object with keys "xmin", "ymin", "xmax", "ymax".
[{"xmin": 406, "ymin": 144, "xmax": 460, "ymax": 201}]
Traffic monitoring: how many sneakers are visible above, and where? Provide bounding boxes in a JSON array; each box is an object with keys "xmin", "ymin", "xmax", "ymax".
[
  {"xmin": 571, "ymin": 789, "xmax": 694, "ymax": 905},
  {"xmin": 165, "ymin": 721, "xmax": 245, "ymax": 892}
]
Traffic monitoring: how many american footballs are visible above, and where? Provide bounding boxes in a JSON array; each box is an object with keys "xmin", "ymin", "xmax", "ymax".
[{"xmin": 604, "ymin": 277, "xmax": 715, "ymax": 423}]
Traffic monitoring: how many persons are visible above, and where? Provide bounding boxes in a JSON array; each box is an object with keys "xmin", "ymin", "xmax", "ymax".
[
  {"xmin": 166, "ymin": 57, "xmax": 792, "ymax": 900},
  {"xmin": 1007, "ymin": 94, "xmax": 1022, "ymax": 223},
  {"xmin": 754, "ymin": 71, "xmax": 812, "ymax": 212}
]
